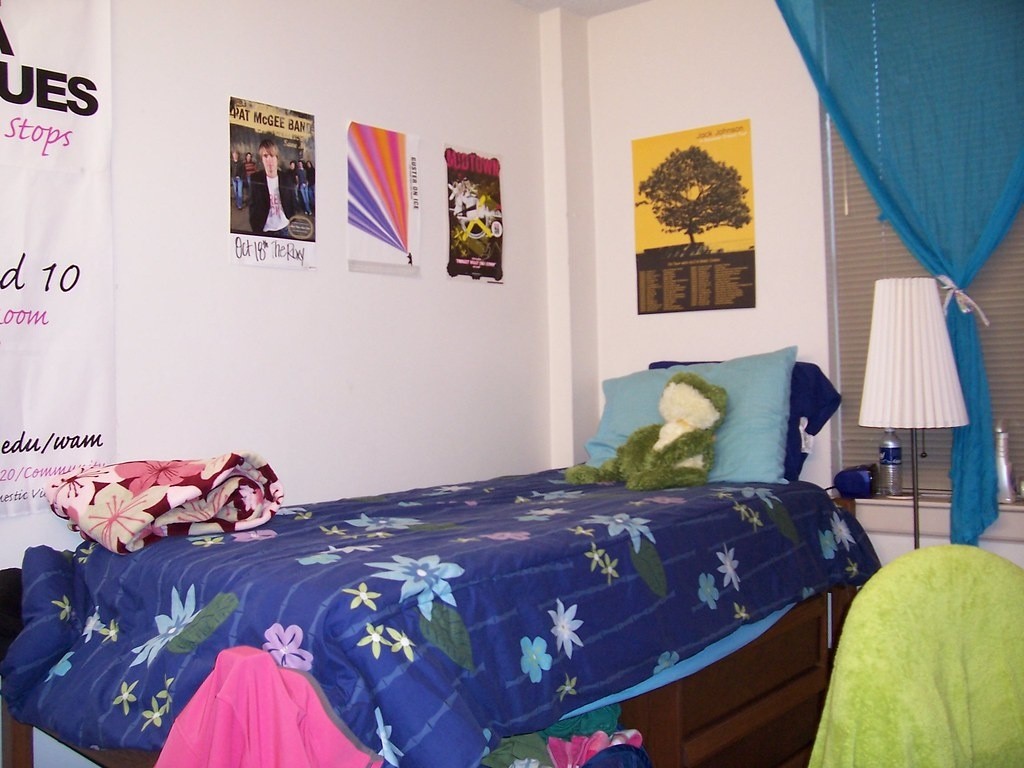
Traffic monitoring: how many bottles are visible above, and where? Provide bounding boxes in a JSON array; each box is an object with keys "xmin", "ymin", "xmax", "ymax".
[
  {"xmin": 880, "ymin": 428, "xmax": 902, "ymax": 496},
  {"xmin": 995, "ymin": 425, "xmax": 1016, "ymax": 503}
]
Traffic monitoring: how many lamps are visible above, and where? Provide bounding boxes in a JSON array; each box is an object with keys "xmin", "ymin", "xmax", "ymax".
[{"xmin": 857, "ymin": 276, "xmax": 971, "ymax": 550}]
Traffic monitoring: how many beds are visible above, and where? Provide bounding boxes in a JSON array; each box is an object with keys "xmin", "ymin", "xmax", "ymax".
[{"xmin": 0, "ymin": 466, "xmax": 883, "ymax": 768}]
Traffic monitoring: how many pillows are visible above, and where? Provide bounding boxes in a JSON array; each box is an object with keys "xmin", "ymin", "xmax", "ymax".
[
  {"xmin": 582, "ymin": 344, "xmax": 800, "ymax": 487},
  {"xmin": 651, "ymin": 357, "xmax": 843, "ymax": 480}
]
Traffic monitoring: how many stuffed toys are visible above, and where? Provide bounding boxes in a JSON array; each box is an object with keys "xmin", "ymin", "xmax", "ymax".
[{"xmin": 563, "ymin": 373, "xmax": 729, "ymax": 489}]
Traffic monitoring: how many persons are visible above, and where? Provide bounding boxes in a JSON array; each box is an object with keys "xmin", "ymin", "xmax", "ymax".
[{"xmin": 230, "ymin": 139, "xmax": 315, "ymax": 239}]
